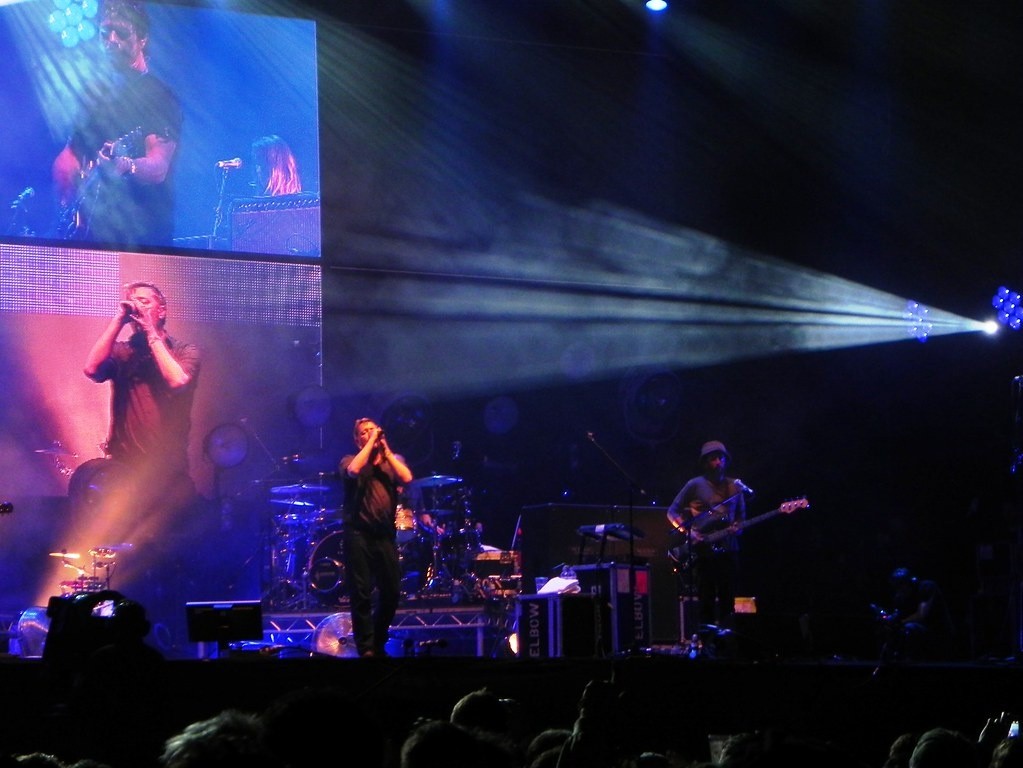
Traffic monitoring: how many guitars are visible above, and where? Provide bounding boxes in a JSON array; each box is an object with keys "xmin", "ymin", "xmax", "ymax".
[
  {"xmin": 666, "ymin": 496, "xmax": 811, "ymax": 565},
  {"xmin": 56, "ymin": 123, "xmax": 146, "ymax": 241}
]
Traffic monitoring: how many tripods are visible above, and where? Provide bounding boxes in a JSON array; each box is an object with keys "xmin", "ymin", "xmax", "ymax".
[{"xmin": 259, "ymin": 492, "xmax": 321, "ymax": 613}]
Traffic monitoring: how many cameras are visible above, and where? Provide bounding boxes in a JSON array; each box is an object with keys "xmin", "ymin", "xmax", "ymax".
[{"xmin": 1008, "ymin": 721, "xmax": 1020, "ymax": 738}]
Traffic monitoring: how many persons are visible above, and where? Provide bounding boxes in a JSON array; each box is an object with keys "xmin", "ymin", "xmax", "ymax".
[
  {"xmin": 17, "ymin": 600, "xmax": 1023, "ymax": 768},
  {"xmin": 392, "ymin": 453, "xmax": 445, "ymax": 534},
  {"xmin": 84, "ymin": 281, "xmax": 200, "ymax": 494},
  {"xmin": 667, "ymin": 441, "xmax": 746, "ymax": 635},
  {"xmin": 338, "ymin": 417, "xmax": 414, "ymax": 658},
  {"xmin": 249, "ymin": 134, "xmax": 302, "ymax": 197},
  {"xmin": 52, "ymin": 0, "xmax": 182, "ymax": 248}
]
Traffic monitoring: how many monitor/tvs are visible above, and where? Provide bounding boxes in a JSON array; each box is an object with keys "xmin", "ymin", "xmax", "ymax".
[{"xmin": 187, "ymin": 599, "xmax": 264, "ymax": 643}]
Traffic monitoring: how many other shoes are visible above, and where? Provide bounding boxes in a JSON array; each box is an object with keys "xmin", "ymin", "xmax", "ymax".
[
  {"xmin": 375, "ymin": 648, "xmax": 393, "ymax": 659},
  {"xmin": 361, "ymin": 649, "xmax": 377, "ymax": 659}
]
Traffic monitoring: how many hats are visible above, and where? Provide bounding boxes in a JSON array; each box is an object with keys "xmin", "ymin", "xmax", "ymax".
[{"xmin": 699, "ymin": 441, "xmax": 731, "ymax": 465}]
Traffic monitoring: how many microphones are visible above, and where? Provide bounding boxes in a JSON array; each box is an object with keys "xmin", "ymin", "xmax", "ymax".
[
  {"xmin": 0, "ymin": 502, "xmax": 14, "ymax": 514},
  {"xmin": 377, "ymin": 431, "xmax": 385, "ymax": 438},
  {"xmin": 13, "ymin": 187, "xmax": 35, "ymax": 204},
  {"xmin": 733, "ymin": 479, "xmax": 754, "ymax": 495},
  {"xmin": 217, "ymin": 158, "xmax": 242, "ymax": 169}
]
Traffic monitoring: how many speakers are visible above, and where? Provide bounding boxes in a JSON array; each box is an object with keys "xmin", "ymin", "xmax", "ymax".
[{"xmin": 227, "ymin": 192, "xmax": 321, "ymax": 257}]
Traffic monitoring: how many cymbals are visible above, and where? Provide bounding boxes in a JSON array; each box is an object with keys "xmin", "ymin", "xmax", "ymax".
[
  {"xmin": 409, "ymin": 475, "xmax": 464, "ymax": 515},
  {"xmin": 268, "ymin": 483, "xmax": 330, "ymax": 512}
]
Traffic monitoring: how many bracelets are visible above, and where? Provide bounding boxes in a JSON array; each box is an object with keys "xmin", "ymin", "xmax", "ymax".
[
  {"xmin": 129, "ymin": 158, "xmax": 135, "ymax": 175},
  {"xmin": 148, "ymin": 336, "xmax": 160, "ymax": 348}
]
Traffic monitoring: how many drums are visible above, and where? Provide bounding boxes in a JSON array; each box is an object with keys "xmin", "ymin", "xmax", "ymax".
[
  {"xmin": 393, "ymin": 503, "xmax": 419, "ymax": 544},
  {"xmin": 307, "ymin": 530, "xmax": 350, "ymax": 607},
  {"xmin": 268, "ymin": 508, "xmax": 333, "ymax": 592}
]
